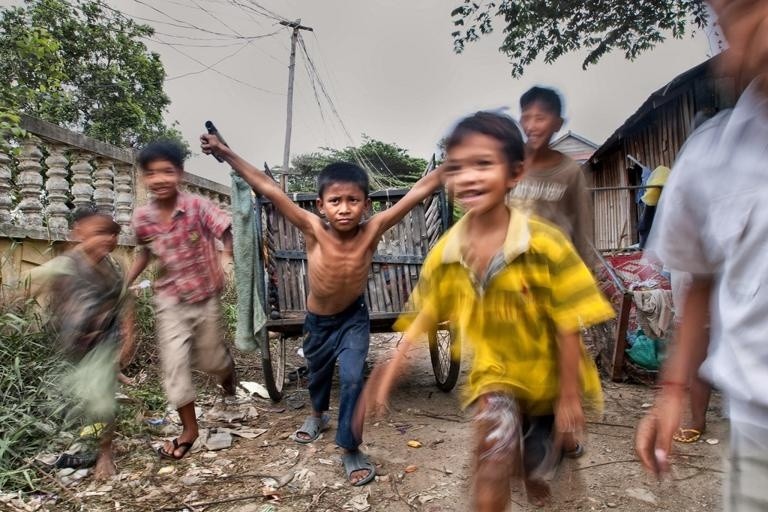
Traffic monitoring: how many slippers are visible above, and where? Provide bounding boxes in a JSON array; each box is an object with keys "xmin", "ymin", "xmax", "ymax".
[
  {"xmin": 157, "ymin": 436, "xmax": 189, "ymax": 461},
  {"xmin": 565, "ymin": 438, "xmax": 583, "ymax": 458},
  {"xmin": 341, "ymin": 451, "xmax": 375, "ymax": 486},
  {"xmin": 294, "ymin": 414, "xmax": 331, "ymax": 443},
  {"xmin": 673, "ymin": 427, "xmax": 702, "ymax": 442}
]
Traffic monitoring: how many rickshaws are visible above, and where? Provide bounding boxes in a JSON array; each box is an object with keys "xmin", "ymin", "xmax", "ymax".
[{"xmin": 199, "ymin": 119, "xmax": 469, "ymax": 406}]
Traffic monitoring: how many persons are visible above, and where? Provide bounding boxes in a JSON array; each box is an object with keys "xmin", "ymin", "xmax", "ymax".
[
  {"xmin": 197, "ymin": 127, "xmax": 443, "ymax": 490},
  {"xmin": 629, "ymin": 0, "xmax": 766, "ymax": 512},
  {"xmin": 21, "ymin": 213, "xmax": 137, "ymax": 485},
  {"xmin": 662, "ymin": 265, "xmax": 722, "ymax": 445},
  {"xmin": 125, "ymin": 140, "xmax": 238, "ymax": 460},
  {"xmin": 501, "ymin": 83, "xmax": 600, "ymax": 459},
  {"xmin": 352, "ymin": 106, "xmax": 620, "ymax": 512}
]
